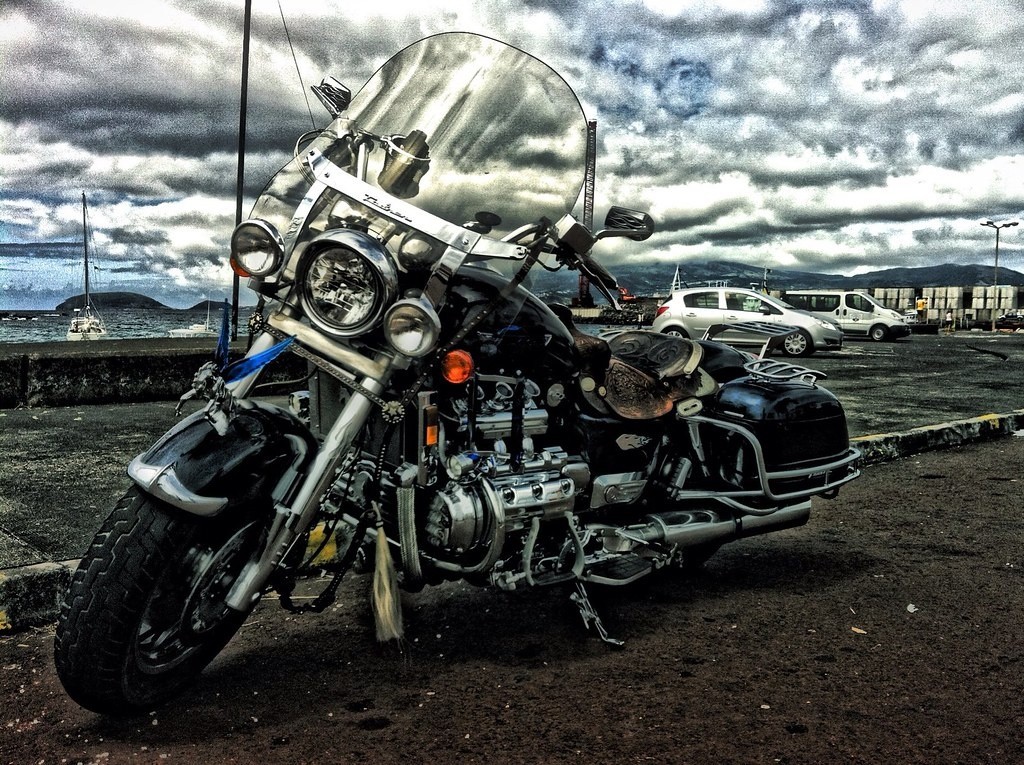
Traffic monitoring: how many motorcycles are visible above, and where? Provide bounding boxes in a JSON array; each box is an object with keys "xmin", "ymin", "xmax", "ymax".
[{"xmin": 51, "ymin": 30, "xmax": 862, "ymax": 704}]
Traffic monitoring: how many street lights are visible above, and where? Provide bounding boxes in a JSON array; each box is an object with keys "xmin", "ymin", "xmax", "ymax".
[{"xmin": 979, "ymin": 221, "xmax": 1019, "ymax": 332}]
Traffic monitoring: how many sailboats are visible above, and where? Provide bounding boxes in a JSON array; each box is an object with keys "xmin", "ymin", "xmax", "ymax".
[
  {"xmin": 167, "ymin": 288, "xmax": 220, "ymax": 338},
  {"xmin": 65, "ymin": 187, "xmax": 112, "ymax": 340}
]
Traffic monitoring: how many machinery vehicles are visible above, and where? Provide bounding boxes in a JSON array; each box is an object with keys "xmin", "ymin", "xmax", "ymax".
[{"xmin": 617, "ymin": 287, "xmax": 637, "ymax": 304}]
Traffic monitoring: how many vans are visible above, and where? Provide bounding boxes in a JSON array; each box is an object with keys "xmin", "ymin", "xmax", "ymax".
[{"xmin": 781, "ymin": 291, "xmax": 911, "ymax": 343}]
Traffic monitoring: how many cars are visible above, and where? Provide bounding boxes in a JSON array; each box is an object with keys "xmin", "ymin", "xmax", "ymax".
[
  {"xmin": 651, "ymin": 287, "xmax": 845, "ymax": 359},
  {"xmin": 998, "ymin": 311, "xmax": 1024, "ymax": 322}
]
